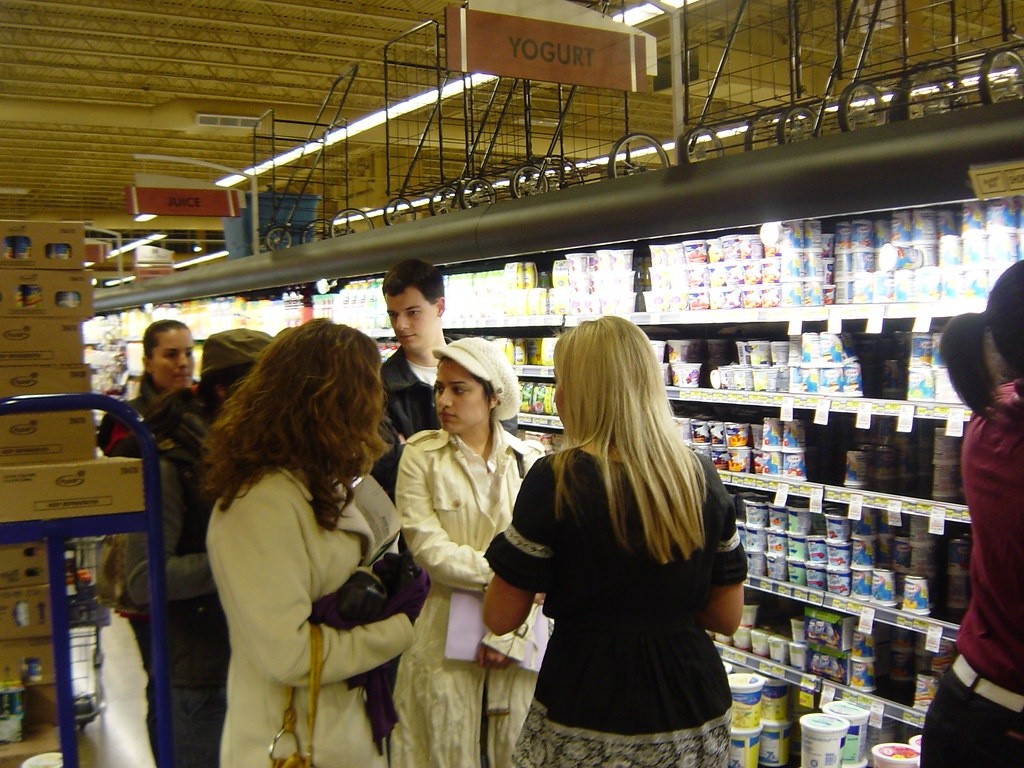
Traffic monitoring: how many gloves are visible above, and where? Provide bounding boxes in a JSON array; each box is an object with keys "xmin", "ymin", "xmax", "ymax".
[{"xmin": 332, "ymin": 533, "xmax": 420, "ymax": 620}]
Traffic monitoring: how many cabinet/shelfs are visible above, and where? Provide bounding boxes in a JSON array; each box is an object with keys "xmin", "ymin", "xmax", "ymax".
[{"xmin": 89, "ymin": 192, "xmax": 1024, "ymax": 766}]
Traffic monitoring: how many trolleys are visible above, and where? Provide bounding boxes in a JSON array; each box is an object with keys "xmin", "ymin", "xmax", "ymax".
[
  {"xmin": 0, "ymin": 388, "xmax": 178, "ymax": 768},
  {"xmin": 61, "ymin": 534, "xmax": 114, "ymax": 734}
]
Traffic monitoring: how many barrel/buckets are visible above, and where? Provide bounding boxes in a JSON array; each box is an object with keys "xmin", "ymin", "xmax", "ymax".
[
  {"xmin": 95, "ymin": 592, "xmax": 111, "ymax": 627},
  {"xmin": 239, "ymin": 189, "xmax": 276, "ymax": 257},
  {"xmin": 21, "ymin": 752, "xmax": 64, "ymax": 768},
  {"xmin": 223, "ymin": 214, "xmax": 250, "ymax": 260},
  {"xmin": 258, "ymin": 189, "xmax": 322, "ymax": 250}
]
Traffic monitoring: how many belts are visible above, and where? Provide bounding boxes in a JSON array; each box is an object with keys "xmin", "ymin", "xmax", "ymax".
[{"xmin": 951, "ymin": 656, "xmax": 1024, "ymax": 713}]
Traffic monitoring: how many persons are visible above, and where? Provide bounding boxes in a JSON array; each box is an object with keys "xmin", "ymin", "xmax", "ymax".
[
  {"xmin": 378, "ymin": 261, "xmax": 519, "ymax": 508},
  {"xmin": 484, "ymin": 316, "xmax": 747, "ymax": 768},
  {"xmin": 203, "ymin": 315, "xmax": 415, "ymax": 767},
  {"xmin": 386, "ymin": 338, "xmax": 549, "ymax": 767},
  {"xmin": 120, "ymin": 328, "xmax": 272, "ymax": 767},
  {"xmin": 920, "ymin": 261, "xmax": 1024, "ymax": 767},
  {"xmin": 97, "ymin": 319, "xmax": 198, "ymax": 455}
]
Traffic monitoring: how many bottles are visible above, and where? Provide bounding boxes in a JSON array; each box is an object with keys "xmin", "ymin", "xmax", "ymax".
[
  {"xmin": 311, "ymin": 294, "xmax": 334, "ymax": 322},
  {"xmin": 64, "ymin": 557, "xmax": 99, "ymax": 626},
  {"xmin": 332, "ymin": 277, "xmax": 393, "ymax": 330},
  {"xmin": 280, "ymin": 290, "xmax": 304, "ymax": 332}
]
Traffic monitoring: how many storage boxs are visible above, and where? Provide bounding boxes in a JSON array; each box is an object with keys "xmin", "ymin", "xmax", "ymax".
[{"xmin": 0, "ymin": 221, "xmax": 144, "ymax": 767}]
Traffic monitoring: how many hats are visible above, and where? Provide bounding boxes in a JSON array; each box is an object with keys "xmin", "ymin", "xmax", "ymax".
[
  {"xmin": 202, "ymin": 328, "xmax": 277, "ymax": 375},
  {"xmin": 434, "ymin": 336, "xmax": 523, "ymax": 420}
]
